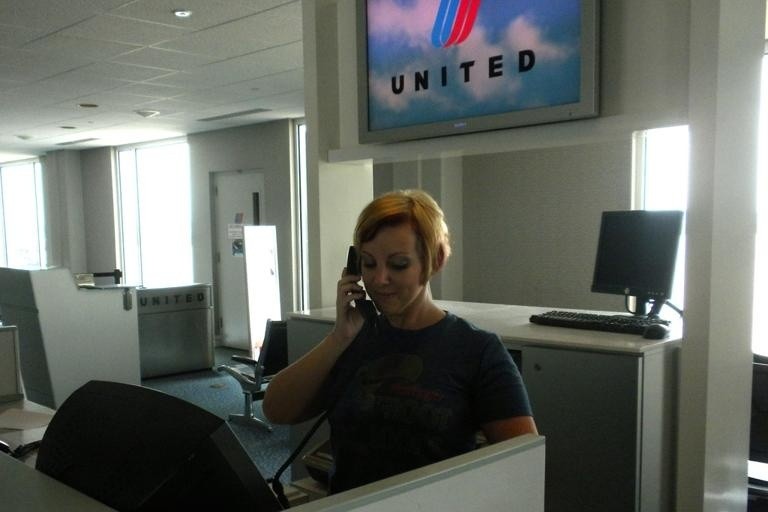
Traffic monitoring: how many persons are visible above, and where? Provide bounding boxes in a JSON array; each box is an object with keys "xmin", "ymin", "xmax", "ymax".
[{"xmin": 262, "ymin": 190, "xmax": 539, "ymax": 495}]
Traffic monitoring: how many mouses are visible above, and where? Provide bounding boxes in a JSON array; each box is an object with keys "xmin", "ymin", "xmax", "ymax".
[{"xmin": 642, "ymin": 324, "xmax": 671, "ymax": 340}]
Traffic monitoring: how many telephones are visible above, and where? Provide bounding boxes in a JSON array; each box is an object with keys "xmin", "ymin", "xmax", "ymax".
[{"xmin": 347, "ymin": 246, "xmax": 378, "ymax": 323}]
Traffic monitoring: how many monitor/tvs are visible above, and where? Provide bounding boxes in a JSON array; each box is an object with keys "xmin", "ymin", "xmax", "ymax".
[
  {"xmin": 357, "ymin": 0, "xmax": 601, "ymax": 145},
  {"xmin": 589, "ymin": 210, "xmax": 687, "ymax": 320}
]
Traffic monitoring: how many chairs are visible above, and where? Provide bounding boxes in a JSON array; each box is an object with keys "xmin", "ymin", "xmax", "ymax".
[
  {"xmin": 748, "ymin": 353, "xmax": 768, "ymax": 512},
  {"xmin": 216, "ymin": 319, "xmax": 286, "ymax": 434}
]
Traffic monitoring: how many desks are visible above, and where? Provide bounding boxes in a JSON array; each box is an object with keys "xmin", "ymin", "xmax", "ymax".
[
  {"xmin": 284, "ymin": 294, "xmax": 681, "ymax": 512},
  {"xmin": 0, "ymin": 397, "xmax": 57, "ymax": 471}
]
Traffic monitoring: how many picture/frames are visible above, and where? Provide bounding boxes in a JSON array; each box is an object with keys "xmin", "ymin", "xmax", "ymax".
[{"xmin": 353, "ymin": 0, "xmax": 601, "ymax": 145}]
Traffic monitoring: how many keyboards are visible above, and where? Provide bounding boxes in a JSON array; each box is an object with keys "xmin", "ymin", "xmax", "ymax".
[{"xmin": 530, "ymin": 308, "xmax": 671, "ymax": 341}]
